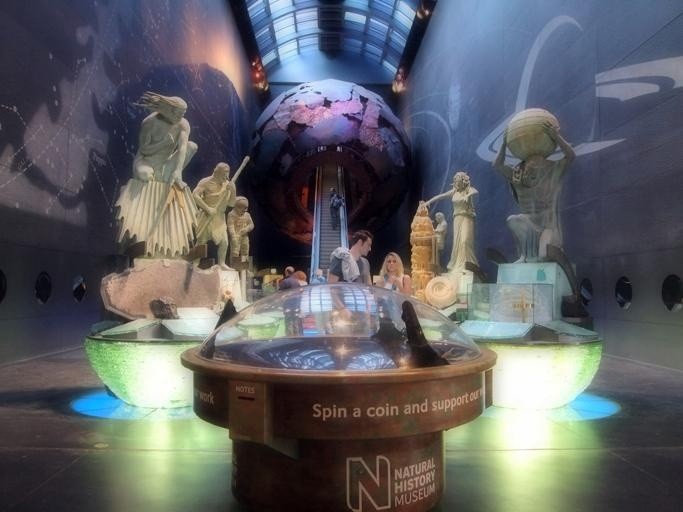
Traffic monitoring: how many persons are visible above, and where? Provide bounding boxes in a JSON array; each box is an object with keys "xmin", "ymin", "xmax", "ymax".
[
  {"xmin": 127, "ymin": 87, "xmax": 197, "ymax": 190},
  {"xmin": 420, "ymin": 169, "xmax": 480, "ymax": 272},
  {"xmin": 329, "ymin": 186, "xmax": 343, "ymax": 230},
  {"xmin": 325, "ymin": 228, "xmax": 375, "ymax": 336},
  {"xmin": 311, "ymin": 267, "xmax": 331, "ymax": 334},
  {"xmin": 277, "ymin": 264, "xmax": 302, "ymax": 339},
  {"xmin": 375, "ymin": 251, "xmax": 410, "ymax": 330},
  {"xmin": 226, "ymin": 196, "xmax": 255, "ymax": 258},
  {"xmin": 433, "ymin": 209, "xmax": 447, "ymax": 251},
  {"xmin": 490, "ymin": 118, "xmax": 578, "ymax": 265},
  {"xmin": 293, "ymin": 270, "xmax": 309, "ymax": 336},
  {"xmin": 191, "ymin": 160, "xmax": 236, "ymax": 273}
]
